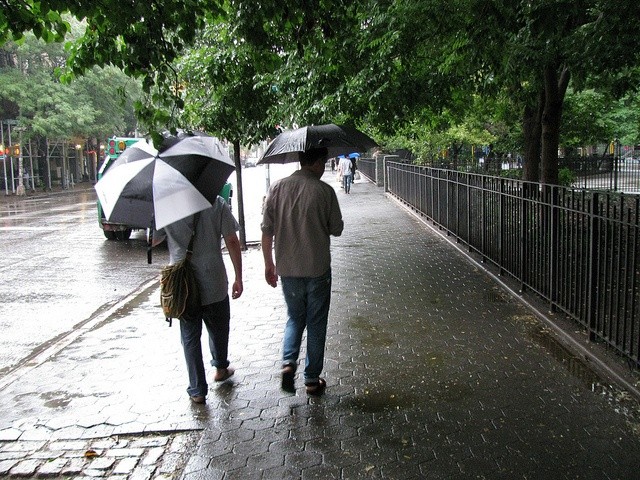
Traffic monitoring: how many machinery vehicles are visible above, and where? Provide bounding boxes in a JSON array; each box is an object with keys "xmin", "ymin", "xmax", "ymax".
[{"xmin": 96, "ymin": 135, "xmax": 233, "ymax": 240}]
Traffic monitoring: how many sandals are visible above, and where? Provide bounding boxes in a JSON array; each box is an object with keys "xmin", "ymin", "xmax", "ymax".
[
  {"xmin": 304, "ymin": 377, "xmax": 326, "ymax": 396},
  {"xmin": 281, "ymin": 362, "xmax": 296, "ymax": 393}
]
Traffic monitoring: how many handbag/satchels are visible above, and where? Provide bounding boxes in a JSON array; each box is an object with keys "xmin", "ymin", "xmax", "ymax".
[{"xmin": 160, "ymin": 260, "xmax": 200, "ymax": 327}]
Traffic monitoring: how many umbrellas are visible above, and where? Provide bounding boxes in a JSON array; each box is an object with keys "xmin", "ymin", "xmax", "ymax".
[
  {"xmin": 93, "ymin": 127, "xmax": 237, "ymax": 264},
  {"xmin": 348, "ymin": 152, "xmax": 361, "ymax": 159},
  {"xmin": 337, "ymin": 153, "xmax": 345, "ymax": 158},
  {"xmin": 256, "ymin": 123, "xmax": 380, "ymax": 165}
]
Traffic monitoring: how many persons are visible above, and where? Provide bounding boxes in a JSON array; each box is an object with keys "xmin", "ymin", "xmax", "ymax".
[
  {"xmin": 349, "ymin": 158, "xmax": 357, "ymax": 184},
  {"xmin": 340, "ymin": 154, "xmax": 353, "ymax": 194},
  {"xmin": 337, "ymin": 158, "xmax": 345, "ymax": 188},
  {"xmin": 164, "ymin": 195, "xmax": 244, "ymax": 403},
  {"xmin": 259, "ymin": 146, "xmax": 344, "ymax": 394}
]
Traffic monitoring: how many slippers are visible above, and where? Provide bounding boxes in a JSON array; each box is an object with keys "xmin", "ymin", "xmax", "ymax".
[
  {"xmin": 189, "ymin": 395, "xmax": 205, "ymax": 404},
  {"xmin": 215, "ymin": 369, "xmax": 234, "ymax": 381}
]
